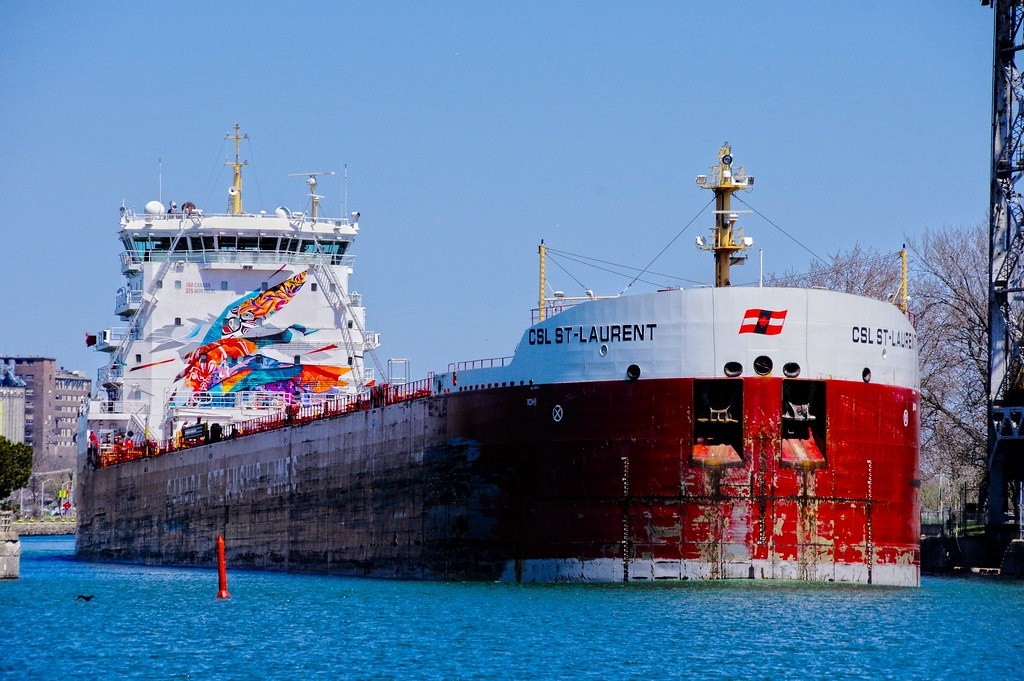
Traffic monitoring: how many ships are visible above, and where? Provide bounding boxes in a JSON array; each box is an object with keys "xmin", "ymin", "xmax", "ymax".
[{"xmin": 73, "ymin": 121, "xmax": 921, "ymax": 590}]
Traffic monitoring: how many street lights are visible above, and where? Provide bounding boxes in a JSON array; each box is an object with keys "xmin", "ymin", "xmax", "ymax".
[
  {"xmin": 60, "ymin": 481, "xmax": 73, "ymax": 517},
  {"xmin": 41, "ymin": 479, "xmax": 54, "ymax": 518}
]
